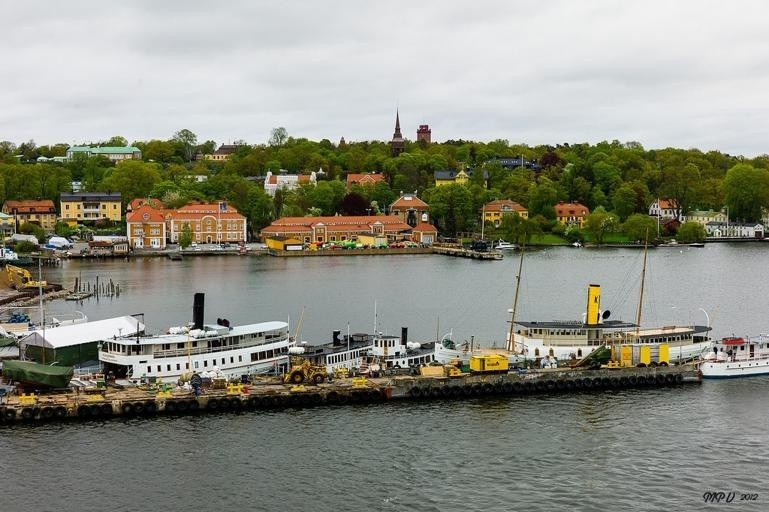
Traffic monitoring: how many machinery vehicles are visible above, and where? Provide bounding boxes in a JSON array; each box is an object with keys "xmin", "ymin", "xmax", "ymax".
[{"xmin": 284, "ymin": 359, "xmax": 327, "ymax": 383}]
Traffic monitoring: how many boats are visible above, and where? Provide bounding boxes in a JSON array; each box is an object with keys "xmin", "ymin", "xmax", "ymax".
[
  {"xmin": 100, "ymin": 293, "xmax": 296, "ymax": 386},
  {"xmin": 506, "ymin": 284, "xmax": 713, "ymax": 368},
  {"xmin": 433, "ymin": 327, "xmax": 527, "ymax": 370},
  {"xmin": 698, "ymin": 332, "xmax": 768, "ymax": 377},
  {"xmin": 354, "ymin": 326, "xmax": 454, "ymax": 371},
  {"xmin": 495, "ymin": 242, "xmax": 520, "ymax": 250},
  {"xmin": 286, "ymin": 328, "xmax": 398, "ymax": 374}
]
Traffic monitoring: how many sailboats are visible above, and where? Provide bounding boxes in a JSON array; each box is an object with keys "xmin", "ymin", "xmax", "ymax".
[{"xmin": 505, "ymin": 224, "xmax": 713, "ymax": 368}]
